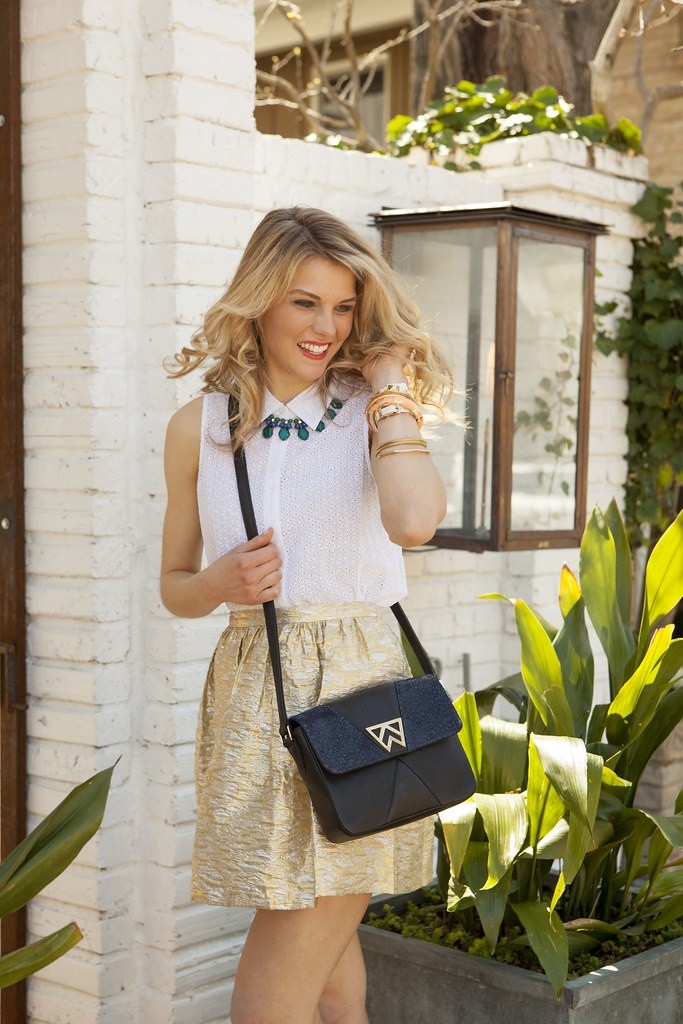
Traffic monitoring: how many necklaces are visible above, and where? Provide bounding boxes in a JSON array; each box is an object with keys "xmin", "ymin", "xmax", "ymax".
[{"xmin": 263, "ymin": 398, "xmax": 344, "ymax": 441}]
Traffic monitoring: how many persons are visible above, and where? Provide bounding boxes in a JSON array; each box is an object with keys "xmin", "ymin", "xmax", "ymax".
[{"xmin": 158, "ymin": 206, "xmax": 447, "ymax": 1024}]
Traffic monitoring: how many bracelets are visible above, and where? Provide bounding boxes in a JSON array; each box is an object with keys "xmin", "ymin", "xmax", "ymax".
[
  {"xmin": 360, "ymin": 381, "xmax": 424, "ymax": 433},
  {"xmin": 378, "ymin": 448, "xmax": 433, "ymax": 457},
  {"xmin": 376, "ymin": 439, "xmax": 429, "ymax": 451}
]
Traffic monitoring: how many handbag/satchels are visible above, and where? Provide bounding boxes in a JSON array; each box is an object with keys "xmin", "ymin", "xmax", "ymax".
[{"xmin": 282, "ymin": 671, "xmax": 477, "ymax": 843}]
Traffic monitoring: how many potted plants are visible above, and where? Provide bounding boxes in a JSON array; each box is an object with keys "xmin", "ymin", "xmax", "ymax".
[
  {"xmin": 355, "ymin": 498, "xmax": 683, "ymax": 1023},
  {"xmin": 382, "ymin": 74, "xmax": 651, "ymax": 178}
]
